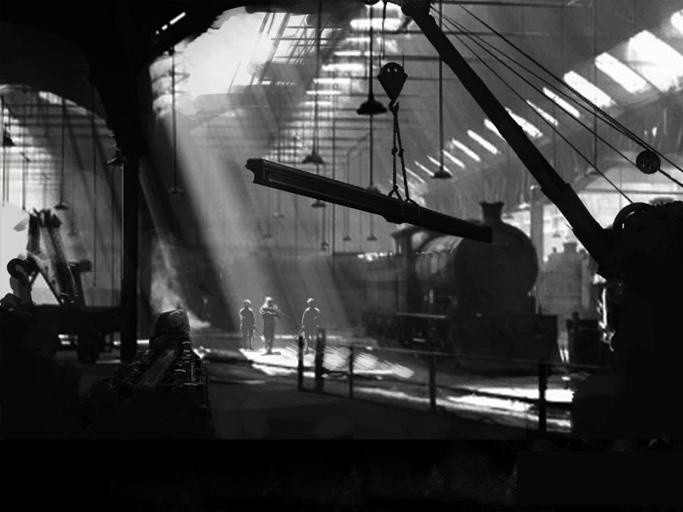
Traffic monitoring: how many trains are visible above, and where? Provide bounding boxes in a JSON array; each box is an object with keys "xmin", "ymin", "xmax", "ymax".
[{"xmin": 308, "ymin": 196, "xmax": 572, "ymax": 378}]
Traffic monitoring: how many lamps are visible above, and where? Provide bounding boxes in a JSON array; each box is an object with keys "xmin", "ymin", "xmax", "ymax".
[
  {"xmin": 1, "ymin": 57, "xmax": 185, "ymax": 244},
  {"xmin": 257, "ymin": 1, "xmax": 682, "ymax": 249}
]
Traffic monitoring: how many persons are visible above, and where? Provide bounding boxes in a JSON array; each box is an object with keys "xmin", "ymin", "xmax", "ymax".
[
  {"xmin": 302, "ymin": 298, "xmax": 320, "ymax": 354},
  {"xmin": 239, "ymin": 299, "xmax": 256, "ymax": 350},
  {"xmin": 259, "ymin": 297, "xmax": 280, "ymax": 356}
]
